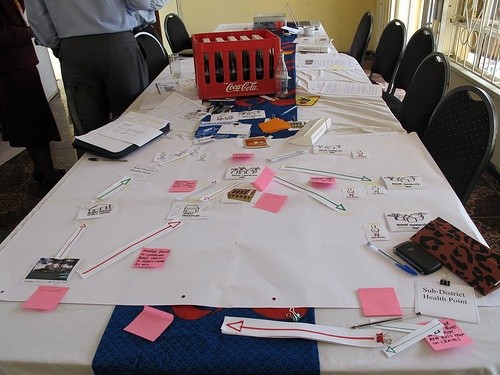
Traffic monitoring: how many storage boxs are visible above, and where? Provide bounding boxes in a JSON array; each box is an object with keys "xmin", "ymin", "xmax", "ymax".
[
  {"xmin": 254, "ymin": 16, "xmax": 287, "ymax": 31},
  {"xmin": 191, "ymin": 29, "xmax": 281, "ymax": 100}
]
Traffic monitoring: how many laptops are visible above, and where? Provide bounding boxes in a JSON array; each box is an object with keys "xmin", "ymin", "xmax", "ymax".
[{"xmin": 287, "ymin": 3, "xmax": 320, "ymax": 28}]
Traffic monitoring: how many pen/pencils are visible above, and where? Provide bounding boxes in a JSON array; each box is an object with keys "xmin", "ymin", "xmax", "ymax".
[
  {"xmin": 367, "ymin": 241, "xmax": 418, "ymax": 275},
  {"xmin": 350, "ymin": 311, "xmax": 421, "ymax": 328},
  {"xmin": 280, "ymin": 105, "xmax": 297, "ymax": 117},
  {"xmin": 88, "ymin": 158, "xmax": 127, "ymax": 162}
]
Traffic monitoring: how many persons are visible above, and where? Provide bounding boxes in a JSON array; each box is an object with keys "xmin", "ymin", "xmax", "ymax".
[
  {"xmin": 0, "ymin": 0, "xmax": 67, "ymax": 183},
  {"xmin": 24, "ymin": 0, "xmax": 169, "ymax": 158}
]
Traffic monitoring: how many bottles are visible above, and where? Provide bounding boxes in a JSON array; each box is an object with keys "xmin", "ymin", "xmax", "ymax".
[{"xmin": 275, "ymin": 51, "xmax": 289, "ymax": 98}]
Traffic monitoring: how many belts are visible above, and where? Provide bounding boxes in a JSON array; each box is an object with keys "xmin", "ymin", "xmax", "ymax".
[{"xmin": 133, "ymin": 23, "xmax": 151, "ymax": 33}]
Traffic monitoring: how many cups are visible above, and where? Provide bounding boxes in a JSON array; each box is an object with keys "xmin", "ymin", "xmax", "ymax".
[{"xmin": 168, "ymin": 53, "xmax": 182, "ymax": 79}]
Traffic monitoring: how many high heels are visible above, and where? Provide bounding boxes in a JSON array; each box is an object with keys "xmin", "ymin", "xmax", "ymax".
[{"xmin": 33, "ymin": 168, "xmax": 65, "ymax": 185}]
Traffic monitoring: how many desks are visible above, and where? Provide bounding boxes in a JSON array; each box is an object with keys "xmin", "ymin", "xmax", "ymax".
[{"xmin": 0, "ymin": 18, "xmax": 500, "ymax": 375}]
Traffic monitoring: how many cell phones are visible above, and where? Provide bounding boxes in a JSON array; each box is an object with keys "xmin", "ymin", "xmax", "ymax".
[{"xmin": 393, "ymin": 241, "xmax": 442, "ymax": 275}]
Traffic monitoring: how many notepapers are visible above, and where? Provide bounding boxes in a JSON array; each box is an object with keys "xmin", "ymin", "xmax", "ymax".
[{"xmin": 357, "ymin": 287, "xmax": 402, "ymax": 316}]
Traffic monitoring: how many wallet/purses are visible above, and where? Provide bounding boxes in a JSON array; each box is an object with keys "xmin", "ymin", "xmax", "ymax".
[{"xmin": 410, "ymin": 216, "xmax": 500, "ymax": 296}]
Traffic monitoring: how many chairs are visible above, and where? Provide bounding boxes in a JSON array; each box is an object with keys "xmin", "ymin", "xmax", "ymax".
[
  {"xmin": 397, "ymin": 52, "xmax": 451, "ymax": 136},
  {"xmin": 420, "ymin": 85, "xmax": 499, "ymax": 205},
  {"xmin": 164, "ymin": 13, "xmax": 193, "ymax": 57},
  {"xmin": 349, "ymin": 10, "xmax": 373, "ymax": 66},
  {"xmin": 369, "ymin": 20, "xmax": 407, "ymax": 84},
  {"xmin": 134, "ymin": 31, "xmax": 168, "ymax": 85},
  {"xmin": 383, "ymin": 27, "xmax": 436, "ymax": 114}
]
